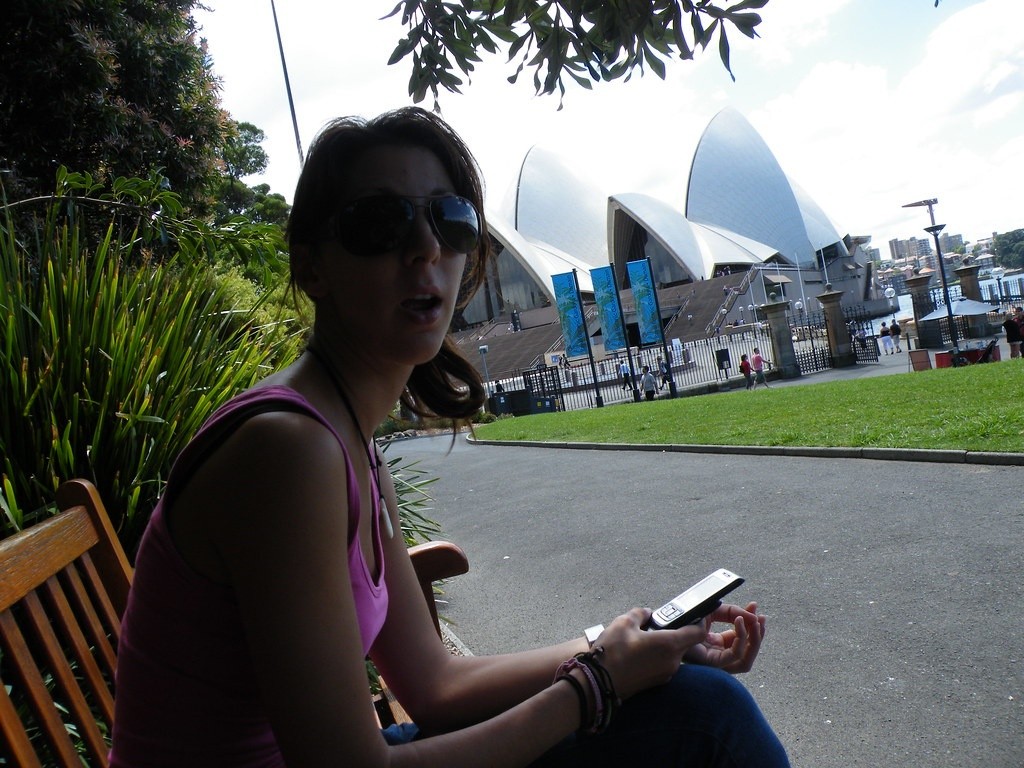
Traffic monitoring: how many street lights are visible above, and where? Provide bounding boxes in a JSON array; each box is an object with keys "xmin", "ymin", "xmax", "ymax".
[
  {"xmin": 748, "ymin": 304, "xmax": 757, "ymax": 340},
  {"xmin": 991, "ymin": 267, "xmax": 1005, "ymax": 299},
  {"xmin": 721, "ymin": 308, "xmax": 728, "ymax": 326},
  {"xmin": 795, "ymin": 301, "xmax": 807, "ymax": 350},
  {"xmin": 885, "ymin": 287, "xmax": 895, "ymax": 319},
  {"xmin": 739, "ymin": 306, "xmax": 744, "ymax": 340},
  {"xmin": 901, "ymin": 197, "xmax": 960, "ymax": 351}
]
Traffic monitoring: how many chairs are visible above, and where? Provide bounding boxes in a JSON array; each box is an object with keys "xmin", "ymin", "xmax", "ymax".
[{"xmin": 0, "ymin": 479, "xmax": 469, "ymax": 768}]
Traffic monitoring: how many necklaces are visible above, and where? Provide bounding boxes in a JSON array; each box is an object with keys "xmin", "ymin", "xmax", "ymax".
[{"xmin": 306, "ymin": 345, "xmax": 394, "ymax": 537}]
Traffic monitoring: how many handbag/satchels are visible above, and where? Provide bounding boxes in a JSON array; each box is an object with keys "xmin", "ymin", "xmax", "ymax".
[{"xmin": 662, "ymin": 366, "xmax": 667, "ymax": 373}]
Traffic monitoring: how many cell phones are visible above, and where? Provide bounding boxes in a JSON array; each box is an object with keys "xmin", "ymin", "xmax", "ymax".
[{"xmin": 641, "ymin": 568, "xmax": 744, "ymax": 632}]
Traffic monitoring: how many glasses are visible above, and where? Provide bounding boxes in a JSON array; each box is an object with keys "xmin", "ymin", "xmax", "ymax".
[
  {"xmin": 1016, "ymin": 310, "xmax": 1020, "ymax": 312},
  {"xmin": 341, "ymin": 188, "xmax": 484, "ymax": 253}
]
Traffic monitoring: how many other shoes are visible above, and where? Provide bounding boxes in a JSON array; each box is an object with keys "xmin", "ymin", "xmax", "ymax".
[
  {"xmin": 629, "ymin": 388, "xmax": 634, "ymax": 391},
  {"xmin": 621, "ymin": 387, "xmax": 626, "ymax": 390},
  {"xmin": 891, "ymin": 352, "xmax": 893, "ymax": 355},
  {"xmin": 659, "ymin": 386, "xmax": 662, "ymax": 390},
  {"xmin": 896, "ymin": 350, "xmax": 902, "ymax": 353}
]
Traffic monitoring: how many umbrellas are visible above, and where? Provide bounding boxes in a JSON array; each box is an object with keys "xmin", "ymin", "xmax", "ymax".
[{"xmin": 917, "ymin": 296, "xmax": 999, "ymax": 338}]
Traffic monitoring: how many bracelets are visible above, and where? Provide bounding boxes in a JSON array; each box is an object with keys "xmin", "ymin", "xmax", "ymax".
[
  {"xmin": 584, "ymin": 623, "xmax": 605, "ymax": 649},
  {"xmin": 553, "ymin": 646, "xmax": 618, "ymax": 738}
]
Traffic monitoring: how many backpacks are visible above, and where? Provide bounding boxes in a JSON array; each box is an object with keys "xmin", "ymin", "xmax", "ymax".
[{"xmin": 739, "ymin": 365, "xmax": 745, "ymax": 373}]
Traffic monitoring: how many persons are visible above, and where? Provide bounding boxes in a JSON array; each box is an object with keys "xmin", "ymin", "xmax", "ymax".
[
  {"xmin": 889, "ymin": 319, "xmax": 902, "ymax": 353},
  {"xmin": 1002, "ymin": 313, "xmax": 1024, "ymax": 358},
  {"xmin": 557, "ymin": 354, "xmax": 571, "ymax": 375},
  {"xmin": 1011, "ymin": 307, "xmax": 1024, "ymax": 358},
  {"xmin": 751, "ymin": 348, "xmax": 774, "ymax": 390},
  {"xmin": 657, "ymin": 356, "xmax": 671, "ymax": 390},
  {"xmin": 740, "ymin": 354, "xmax": 755, "ymax": 390},
  {"xmin": 880, "ymin": 322, "xmax": 894, "ymax": 355},
  {"xmin": 733, "ymin": 320, "xmax": 738, "ymax": 327},
  {"xmin": 495, "ymin": 380, "xmax": 505, "ymax": 392},
  {"xmin": 719, "ymin": 265, "xmax": 731, "ymax": 276},
  {"xmin": 740, "ymin": 318, "xmax": 746, "ymax": 324},
  {"xmin": 109, "ymin": 107, "xmax": 793, "ymax": 768},
  {"xmin": 618, "ymin": 360, "xmax": 633, "ymax": 390},
  {"xmin": 639, "ymin": 365, "xmax": 660, "ymax": 401},
  {"xmin": 855, "ymin": 324, "xmax": 867, "ymax": 349},
  {"xmin": 688, "ymin": 313, "xmax": 693, "ymax": 325},
  {"xmin": 723, "ymin": 285, "xmax": 728, "ymax": 296}
]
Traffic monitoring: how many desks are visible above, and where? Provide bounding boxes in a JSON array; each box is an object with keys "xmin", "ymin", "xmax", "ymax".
[{"xmin": 934, "ymin": 344, "xmax": 1001, "ymax": 369}]
